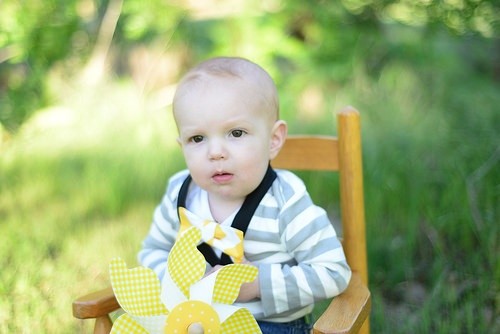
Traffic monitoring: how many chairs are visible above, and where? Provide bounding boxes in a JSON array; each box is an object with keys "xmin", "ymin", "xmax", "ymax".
[{"xmin": 71, "ymin": 105, "xmax": 372, "ymax": 334}]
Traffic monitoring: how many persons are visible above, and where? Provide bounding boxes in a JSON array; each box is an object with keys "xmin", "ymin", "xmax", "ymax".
[{"xmin": 137, "ymin": 56, "xmax": 352, "ymax": 334}]
